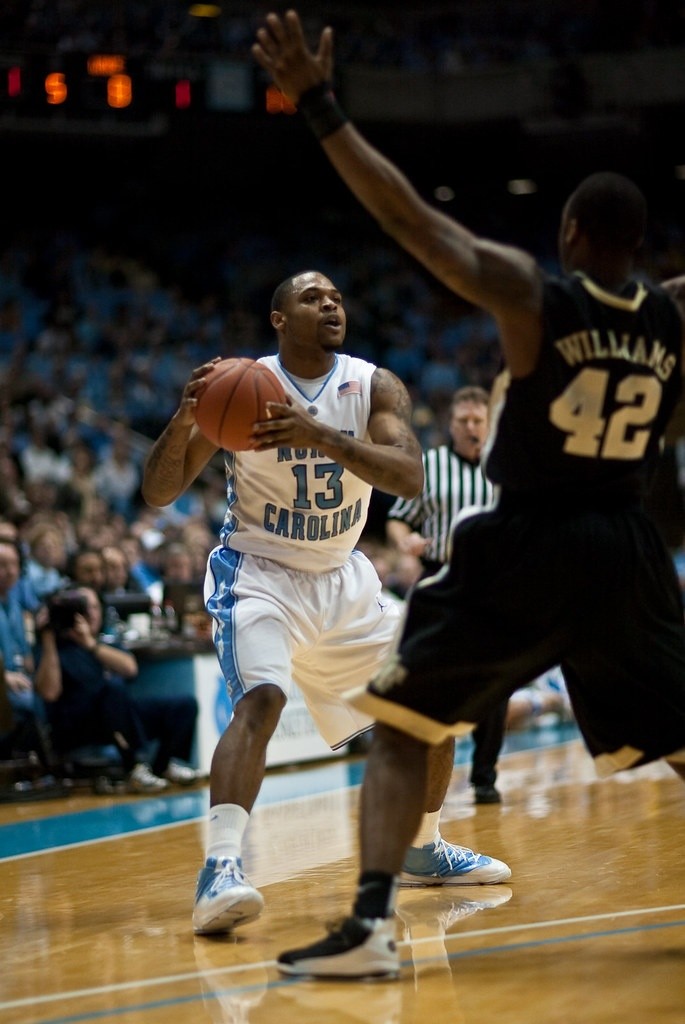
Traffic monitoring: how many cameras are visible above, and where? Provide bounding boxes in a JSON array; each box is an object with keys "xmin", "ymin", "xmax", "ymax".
[{"xmin": 48, "ymin": 593, "xmax": 87, "ymax": 628}]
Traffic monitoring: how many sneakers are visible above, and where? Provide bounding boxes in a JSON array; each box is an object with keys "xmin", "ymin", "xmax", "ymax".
[
  {"xmin": 273, "ymin": 918, "xmax": 401, "ymax": 977},
  {"xmin": 470, "ymin": 782, "xmax": 501, "ymax": 804},
  {"xmin": 191, "ymin": 854, "xmax": 263, "ymax": 937},
  {"xmin": 398, "ymin": 836, "xmax": 513, "ymax": 886}
]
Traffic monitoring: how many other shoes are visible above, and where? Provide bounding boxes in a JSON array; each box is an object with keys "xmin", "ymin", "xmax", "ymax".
[{"xmin": 8, "ymin": 753, "xmax": 197, "ymax": 798}]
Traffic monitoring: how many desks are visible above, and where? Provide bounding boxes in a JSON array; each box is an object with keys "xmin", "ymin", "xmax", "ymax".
[{"xmin": 128, "ymin": 643, "xmax": 349, "ymax": 777}]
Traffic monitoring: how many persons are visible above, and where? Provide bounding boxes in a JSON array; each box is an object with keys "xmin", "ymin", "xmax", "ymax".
[
  {"xmin": 248, "ymin": 12, "xmax": 685, "ymax": 978},
  {"xmin": 503, "ymin": 663, "xmax": 584, "ymax": 738},
  {"xmin": 0, "ymin": 242, "xmax": 239, "ymax": 799},
  {"xmin": 241, "ymin": 285, "xmax": 504, "ymax": 599},
  {"xmin": 140, "ymin": 268, "xmax": 512, "ymax": 935},
  {"xmin": 387, "ymin": 384, "xmax": 508, "ymax": 807}
]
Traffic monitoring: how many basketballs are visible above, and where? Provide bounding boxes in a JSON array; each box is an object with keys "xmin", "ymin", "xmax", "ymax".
[{"xmin": 190, "ymin": 358, "xmax": 284, "ymax": 452}]
